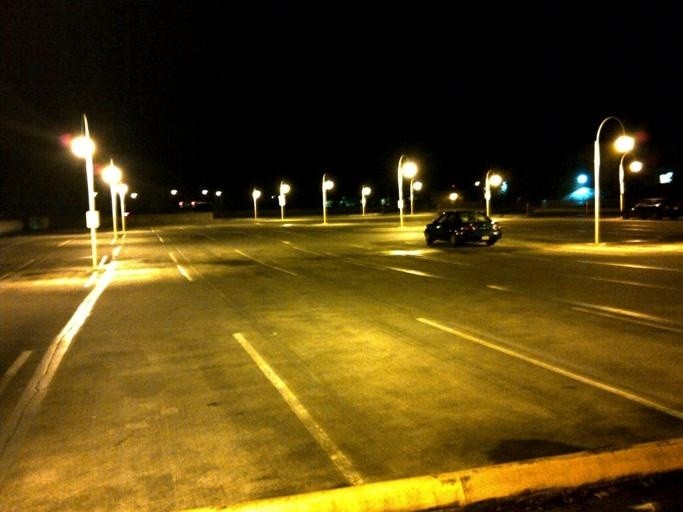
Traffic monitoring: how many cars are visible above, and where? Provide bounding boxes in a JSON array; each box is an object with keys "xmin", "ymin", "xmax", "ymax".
[
  {"xmin": 630, "ymin": 197, "xmax": 681, "ymax": 219},
  {"xmin": 424, "ymin": 210, "xmax": 501, "ymax": 248}
]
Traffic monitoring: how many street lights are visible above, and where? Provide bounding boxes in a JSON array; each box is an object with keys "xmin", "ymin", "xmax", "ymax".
[
  {"xmin": 70, "ymin": 113, "xmax": 128, "ymax": 271},
  {"xmin": 618, "ymin": 152, "xmax": 642, "ymax": 215},
  {"xmin": 251, "ymin": 187, "xmax": 261, "ymax": 219},
  {"xmin": 361, "ymin": 185, "xmax": 370, "ymax": 216},
  {"xmin": 593, "ymin": 115, "xmax": 633, "ymax": 245},
  {"xmin": 396, "ymin": 153, "xmax": 422, "ymax": 227},
  {"xmin": 321, "ymin": 174, "xmax": 334, "ymax": 223},
  {"xmin": 279, "ymin": 181, "xmax": 289, "ymax": 220},
  {"xmin": 409, "ymin": 178, "xmax": 424, "ymax": 215},
  {"xmin": 484, "ymin": 169, "xmax": 502, "ymax": 217}
]
